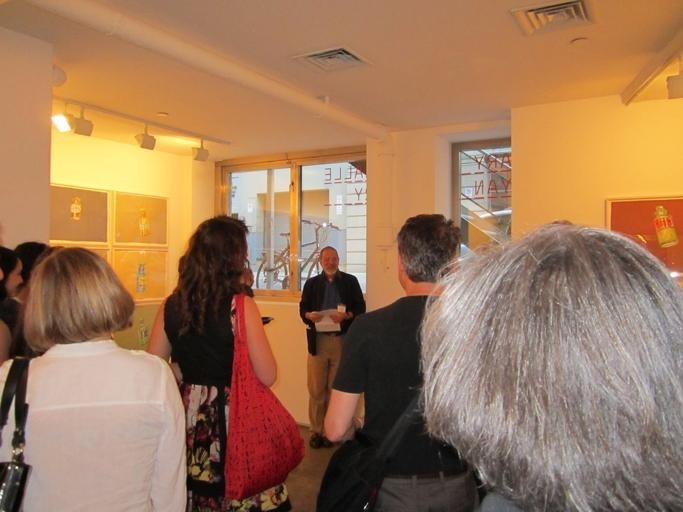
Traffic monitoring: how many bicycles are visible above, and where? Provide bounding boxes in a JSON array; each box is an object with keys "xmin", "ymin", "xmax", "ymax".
[{"xmin": 253, "ymin": 217, "xmax": 345, "ymax": 293}]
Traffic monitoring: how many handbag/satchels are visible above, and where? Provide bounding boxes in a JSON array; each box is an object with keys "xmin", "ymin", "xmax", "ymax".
[
  {"xmin": 0, "ymin": 461, "xmax": 30, "ymax": 512},
  {"xmin": 223, "ymin": 335, "xmax": 305, "ymax": 501},
  {"xmin": 316, "ymin": 439, "xmax": 388, "ymax": 512}
]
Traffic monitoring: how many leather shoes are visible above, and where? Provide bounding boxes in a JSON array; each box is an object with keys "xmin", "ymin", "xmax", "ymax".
[{"xmin": 310, "ymin": 433, "xmax": 332, "ymax": 448}]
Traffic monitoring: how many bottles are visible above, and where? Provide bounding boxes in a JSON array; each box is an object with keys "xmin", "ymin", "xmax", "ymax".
[
  {"xmin": 652, "ymin": 204, "xmax": 678, "ymax": 249},
  {"xmin": 137, "ymin": 316, "xmax": 148, "ymax": 345},
  {"xmin": 136, "ymin": 263, "xmax": 146, "ymax": 293}
]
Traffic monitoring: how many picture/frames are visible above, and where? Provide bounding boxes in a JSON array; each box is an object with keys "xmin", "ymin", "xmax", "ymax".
[
  {"xmin": 605, "ymin": 197, "xmax": 683, "ymax": 288},
  {"xmin": 48, "ymin": 182, "xmax": 169, "ymax": 353}
]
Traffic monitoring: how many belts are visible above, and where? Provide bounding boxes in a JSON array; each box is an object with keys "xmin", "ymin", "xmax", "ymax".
[
  {"xmin": 323, "ymin": 330, "xmax": 342, "ymax": 336},
  {"xmin": 389, "ymin": 466, "xmax": 469, "ymax": 480}
]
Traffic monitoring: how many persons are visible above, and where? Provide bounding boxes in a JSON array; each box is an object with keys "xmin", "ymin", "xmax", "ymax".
[
  {"xmin": 14, "ymin": 242, "xmax": 50, "ymax": 283},
  {"xmin": 324, "ymin": 212, "xmax": 482, "ymax": 512},
  {"xmin": 0, "ymin": 244, "xmax": 25, "ymax": 367},
  {"xmin": 0, "ymin": 246, "xmax": 187, "ymax": 511},
  {"xmin": 416, "ymin": 219, "xmax": 682, "ymax": 512},
  {"xmin": 297, "ymin": 245, "xmax": 365, "ymax": 450},
  {"xmin": 150, "ymin": 212, "xmax": 295, "ymax": 511}
]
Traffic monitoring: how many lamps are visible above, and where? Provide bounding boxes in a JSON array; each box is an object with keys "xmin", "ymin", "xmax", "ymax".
[
  {"xmin": 620, "ymin": 28, "xmax": 683, "ymax": 106},
  {"xmin": 51, "ymin": 96, "xmax": 232, "ymax": 163}
]
